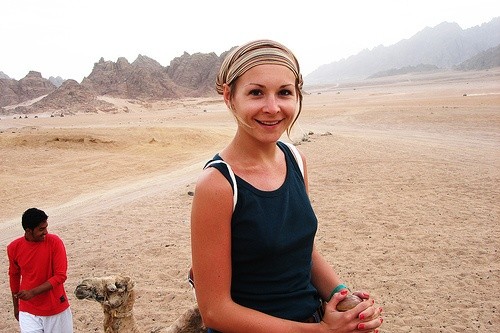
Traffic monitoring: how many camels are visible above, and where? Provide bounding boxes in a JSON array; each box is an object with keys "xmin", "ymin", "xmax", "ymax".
[{"xmin": 74, "ymin": 274, "xmax": 205, "ymax": 333}]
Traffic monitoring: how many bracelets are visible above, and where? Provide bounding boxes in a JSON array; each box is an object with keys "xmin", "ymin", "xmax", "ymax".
[{"xmin": 326, "ymin": 284, "xmax": 352, "ymax": 304}]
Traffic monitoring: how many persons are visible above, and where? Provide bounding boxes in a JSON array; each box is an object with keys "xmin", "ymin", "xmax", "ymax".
[
  {"xmin": 191, "ymin": 39, "xmax": 384, "ymax": 333},
  {"xmin": 7, "ymin": 208, "xmax": 73, "ymax": 333}
]
